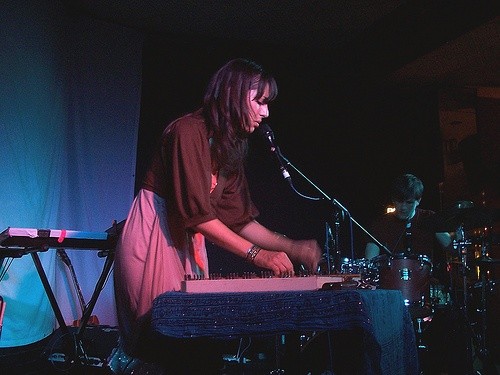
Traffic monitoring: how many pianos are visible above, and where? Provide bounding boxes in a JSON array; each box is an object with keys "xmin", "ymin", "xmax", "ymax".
[{"xmin": 0, "ymin": 226, "xmax": 110, "ymax": 250}]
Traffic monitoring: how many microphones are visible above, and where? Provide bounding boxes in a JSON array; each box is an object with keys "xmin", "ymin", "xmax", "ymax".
[
  {"xmin": 260, "ymin": 124, "xmax": 292, "ymax": 184},
  {"xmin": 406, "ymin": 219, "xmax": 412, "ymax": 252}
]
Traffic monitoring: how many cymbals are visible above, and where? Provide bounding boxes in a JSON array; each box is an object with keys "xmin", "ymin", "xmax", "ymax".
[{"xmin": 470, "ymin": 256, "xmax": 500, "ymax": 266}]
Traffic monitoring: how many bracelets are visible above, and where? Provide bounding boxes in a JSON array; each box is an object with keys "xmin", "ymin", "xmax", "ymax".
[{"xmin": 245, "ymin": 244, "xmax": 264, "ymax": 266}]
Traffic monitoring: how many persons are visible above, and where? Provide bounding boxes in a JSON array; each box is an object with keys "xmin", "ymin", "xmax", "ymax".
[
  {"xmin": 364, "ymin": 173, "xmax": 454, "ymax": 259},
  {"xmin": 112, "ymin": 56, "xmax": 324, "ymax": 375}
]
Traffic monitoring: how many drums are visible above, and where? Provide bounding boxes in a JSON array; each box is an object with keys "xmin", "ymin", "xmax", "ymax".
[
  {"xmin": 440, "ymin": 255, "xmax": 493, "ymax": 292},
  {"xmin": 323, "ymin": 257, "xmax": 373, "ymax": 288},
  {"xmin": 297, "ymin": 328, "xmax": 363, "ymax": 375},
  {"xmin": 370, "ymin": 252, "xmax": 433, "ymax": 318}
]
